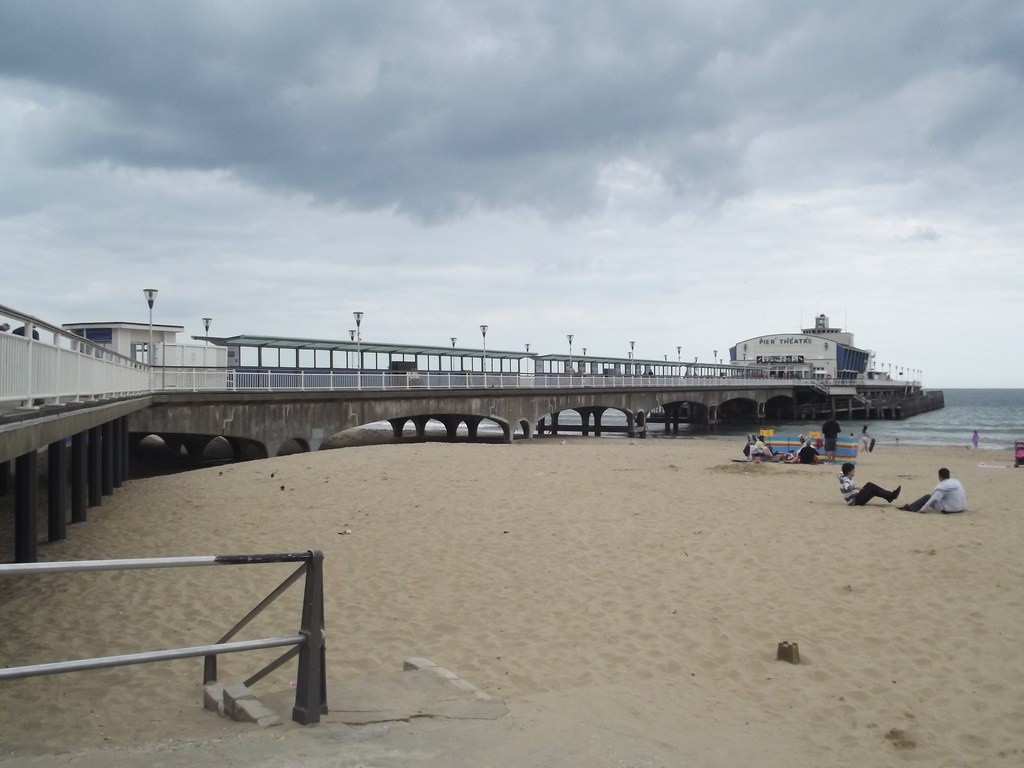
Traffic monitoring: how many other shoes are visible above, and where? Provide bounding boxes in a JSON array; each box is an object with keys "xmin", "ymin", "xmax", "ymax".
[{"xmin": 887, "ymin": 486, "xmax": 901, "ymax": 503}]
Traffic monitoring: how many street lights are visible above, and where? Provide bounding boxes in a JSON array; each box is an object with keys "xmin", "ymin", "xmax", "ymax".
[
  {"xmin": 450, "ymin": 337, "xmax": 458, "ymax": 371},
  {"xmin": 629, "ymin": 341, "xmax": 636, "ymax": 359},
  {"xmin": 348, "ymin": 329, "xmax": 357, "ymax": 387},
  {"xmin": 695, "ymin": 357, "xmax": 698, "ymax": 362},
  {"xmin": 525, "ymin": 343, "xmax": 531, "ymax": 384},
  {"xmin": 582, "ymin": 346, "xmax": 588, "ymax": 386},
  {"xmin": 352, "ymin": 311, "xmax": 364, "ymax": 391},
  {"xmin": 480, "ymin": 324, "xmax": 489, "ymax": 389},
  {"xmin": 567, "ymin": 334, "xmax": 574, "ymax": 387},
  {"xmin": 714, "ymin": 350, "xmax": 718, "ymax": 364},
  {"xmin": 719, "ymin": 358, "xmax": 723, "ymax": 364},
  {"xmin": 664, "ymin": 354, "xmax": 668, "ymax": 360},
  {"xmin": 143, "ymin": 288, "xmax": 158, "ymax": 394},
  {"xmin": 627, "ymin": 351, "xmax": 633, "ymax": 376},
  {"xmin": 201, "ymin": 316, "xmax": 213, "ymax": 387},
  {"xmin": 676, "ymin": 346, "xmax": 682, "ymax": 375}
]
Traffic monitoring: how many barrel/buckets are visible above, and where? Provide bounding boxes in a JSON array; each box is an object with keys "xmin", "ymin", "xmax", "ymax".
[{"xmin": 755, "ymin": 456, "xmax": 761, "ymax": 463}]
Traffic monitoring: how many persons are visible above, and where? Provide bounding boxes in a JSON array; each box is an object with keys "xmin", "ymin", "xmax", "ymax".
[
  {"xmin": 0, "ymin": 322, "xmax": 10, "ymax": 332},
  {"xmin": 685, "ymin": 371, "xmax": 689, "ymax": 376},
  {"xmin": 720, "ymin": 373, "xmax": 725, "ymax": 379},
  {"xmin": 649, "ymin": 369, "xmax": 653, "ymax": 375},
  {"xmin": 972, "ymin": 430, "xmax": 980, "ymax": 448},
  {"xmin": 643, "ymin": 372, "xmax": 647, "ymax": 375},
  {"xmin": 11, "ymin": 323, "xmax": 40, "ymax": 340},
  {"xmin": 784, "ymin": 437, "xmax": 820, "ymax": 464},
  {"xmin": 897, "ymin": 467, "xmax": 968, "ymax": 514},
  {"xmin": 838, "ymin": 463, "xmax": 901, "ymax": 506},
  {"xmin": 860, "ymin": 425, "xmax": 871, "ymax": 453},
  {"xmin": 755, "ymin": 435, "xmax": 766, "ymax": 448},
  {"xmin": 821, "ymin": 415, "xmax": 841, "ymax": 463},
  {"xmin": 695, "ymin": 372, "xmax": 697, "ymax": 376},
  {"xmin": 850, "ymin": 433, "xmax": 854, "ymax": 438}
]
